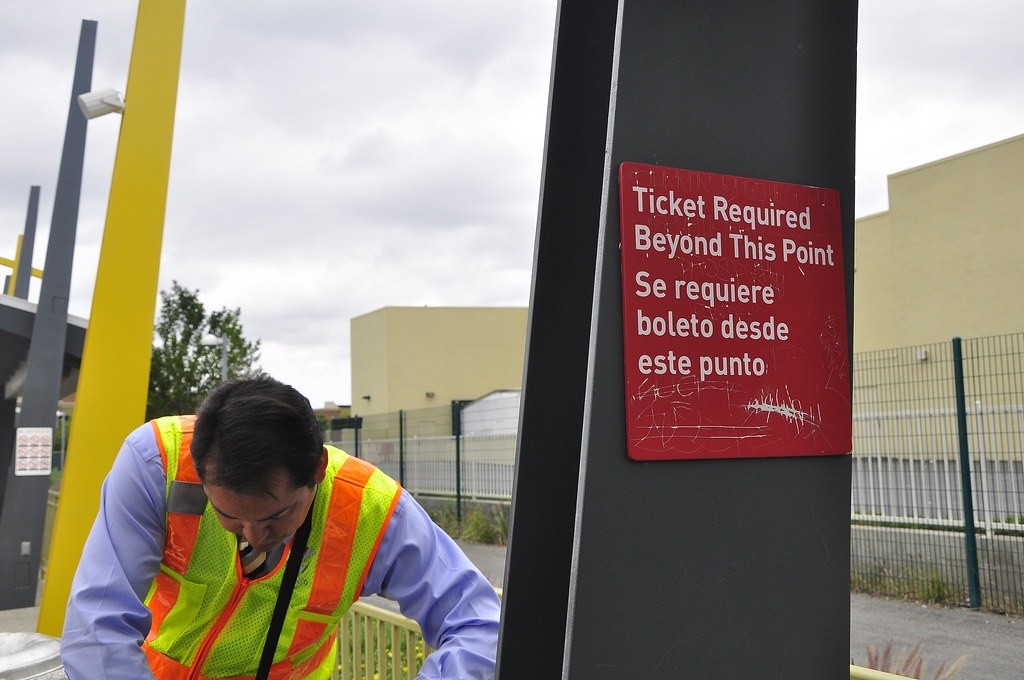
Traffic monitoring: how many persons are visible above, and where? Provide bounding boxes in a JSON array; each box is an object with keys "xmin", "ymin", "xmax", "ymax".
[{"xmin": 60, "ymin": 372, "xmax": 501, "ymax": 680}]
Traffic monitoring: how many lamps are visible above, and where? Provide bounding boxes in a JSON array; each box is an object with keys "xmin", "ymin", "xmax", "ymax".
[
  {"xmin": 917, "ymin": 352, "xmax": 927, "ymax": 361},
  {"xmin": 426, "ymin": 391, "xmax": 435, "ymax": 397}
]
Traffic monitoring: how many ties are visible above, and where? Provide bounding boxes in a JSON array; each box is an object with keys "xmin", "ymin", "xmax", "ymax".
[{"xmin": 238, "ymin": 534, "xmax": 267, "ymax": 582}]
